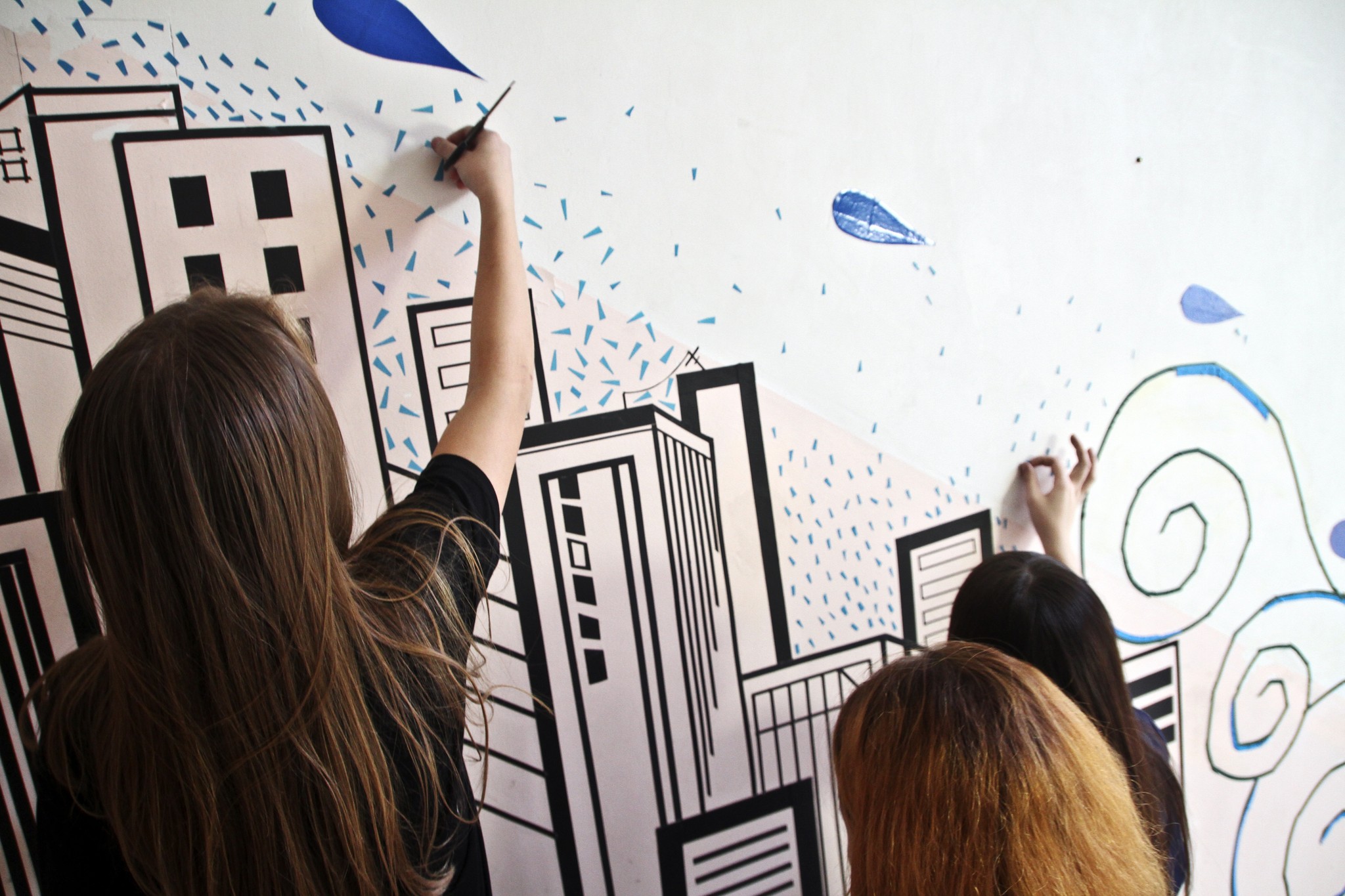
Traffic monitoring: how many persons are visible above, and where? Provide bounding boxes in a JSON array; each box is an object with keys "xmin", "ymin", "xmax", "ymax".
[
  {"xmin": 948, "ymin": 432, "xmax": 1192, "ymax": 896},
  {"xmin": 828, "ymin": 643, "xmax": 1170, "ymax": 896},
  {"xmin": 26, "ymin": 125, "xmax": 532, "ymax": 896}
]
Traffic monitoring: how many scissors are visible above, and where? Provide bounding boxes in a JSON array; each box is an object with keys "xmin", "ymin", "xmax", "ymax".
[{"xmin": 444, "ymin": 82, "xmax": 512, "ymax": 169}]
